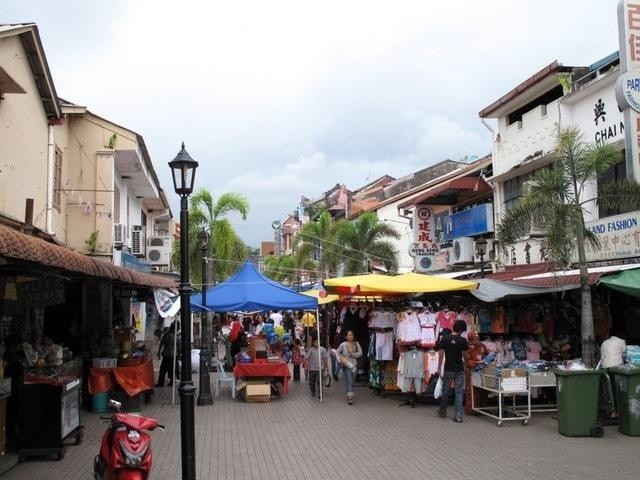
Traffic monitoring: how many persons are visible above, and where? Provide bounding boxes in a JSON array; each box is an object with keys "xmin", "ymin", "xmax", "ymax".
[
  {"xmin": 153, "ymin": 320, "xmax": 181, "ymax": 387},
  {"xmin": 213, "ymin": 307, "xmax": 364, "ymax": 405},
  {"xmin": 465, "ymin": 329, "xmax": 488, "ymax": 415},
  {"xmin": 598, "ymin": 324, "xmax": 628, "ymax": 420},
  {"xmin": 433, "ymin": 318, "xmax": 467, "ymax": 425}
]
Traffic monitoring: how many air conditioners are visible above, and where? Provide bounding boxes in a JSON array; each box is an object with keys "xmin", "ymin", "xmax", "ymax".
[
  {"xmin": 445, "ymin": 237, "xmax": 474, "ymax": 266},
  {"xmin": 114, "ymin": 224, "xmax": 172, "ymax": 265}
]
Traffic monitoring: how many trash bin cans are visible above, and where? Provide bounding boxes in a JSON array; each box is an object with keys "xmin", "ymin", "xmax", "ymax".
[
  {"xmin": 607, "ymin": 363, "xmax": 640, "ymax": 436},
  {"xmin": 551, "ymin": 364, "xmax": 607, "ymax": 438}
]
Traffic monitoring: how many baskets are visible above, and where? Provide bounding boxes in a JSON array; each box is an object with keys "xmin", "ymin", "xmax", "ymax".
[{"xmin": 92, "ymin": 357, "xmax": 118, "ymax": 369}]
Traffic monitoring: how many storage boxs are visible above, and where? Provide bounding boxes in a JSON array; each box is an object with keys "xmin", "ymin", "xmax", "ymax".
[{"xmin": 236, "ymin": 381, "xmax": 278, "ymax": 402}]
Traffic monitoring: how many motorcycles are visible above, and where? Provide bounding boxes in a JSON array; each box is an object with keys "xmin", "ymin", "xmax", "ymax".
[{"xmin": 92, "ymin": 398, "xmax": 166, "ymax": 480}]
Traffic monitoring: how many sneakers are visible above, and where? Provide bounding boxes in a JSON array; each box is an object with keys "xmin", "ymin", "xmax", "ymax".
[
  {"xmin": 435, "ymin": 408, "xmax": 447, "ymax": 418},
  {"xmin": 344, "ymin": 393, "xmax": 353, "ymax": 404},
  {"xmin": 155, "ymin": 382, "xmax": 173, "ymax": 387},
  {"xmin": 452, "ymin": 415, "xmax": 462, "ymax": 423},
  {"xmin": 312, "ymin": 391, "xmax": 320, "ymax": 399}
]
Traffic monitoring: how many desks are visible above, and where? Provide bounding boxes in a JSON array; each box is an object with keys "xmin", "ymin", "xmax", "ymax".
[{"xmin": 234, "ymin": 352, "xmax": 288, "ymax": 397}]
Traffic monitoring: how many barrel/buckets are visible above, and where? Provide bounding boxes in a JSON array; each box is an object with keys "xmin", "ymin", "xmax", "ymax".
[
  {"xmin": 90, "ymin": 391, "xmax": 111, "ymax": 413},
  {"xmin": 191, "ymin": 349, "xmax": 200, "ymax": 373}
]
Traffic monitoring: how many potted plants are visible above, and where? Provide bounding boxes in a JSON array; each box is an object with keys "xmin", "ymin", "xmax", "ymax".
[{"xmin": 84, "ymin": 230, "xmax": 100, "ymax": 254}]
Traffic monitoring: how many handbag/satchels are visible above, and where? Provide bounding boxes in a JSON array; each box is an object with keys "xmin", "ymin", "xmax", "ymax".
[{"xmin": 239, "ymin": 331, "xmax": 247, "ymax": 343}]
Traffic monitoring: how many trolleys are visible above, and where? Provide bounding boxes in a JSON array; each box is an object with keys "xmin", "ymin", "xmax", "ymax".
[
  {"xmin": 11, "ymin": 363, "xmax": 86, "ymax": 461},
  {"xmin": 469, "ymin": 370, "xmax": 532, "ymax": 428},
  {"xmin": 502, "ymin": 373, "xmax": 561, "ymax": 420}
]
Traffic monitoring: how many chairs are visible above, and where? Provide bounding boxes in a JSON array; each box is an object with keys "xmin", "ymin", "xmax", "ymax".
[{"xmin": 214, "ymin": 358, "xmax": 235, "ymax": 399}]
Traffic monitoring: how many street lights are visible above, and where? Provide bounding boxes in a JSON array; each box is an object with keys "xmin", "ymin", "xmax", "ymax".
[
  {"xmin": 476, "ymin": 233, "xmax": 490, "ymax": 278},
  {"xmin": 385, "ymin": 257, "xmax": 393, "ymax": 273},
  {"xmin": 194, "ymin": 223, "xmax": 217, "ymax": 405},
  {"xmin": 168, "ymin": 141, "xmax": 202, "ymax": 480}
]
